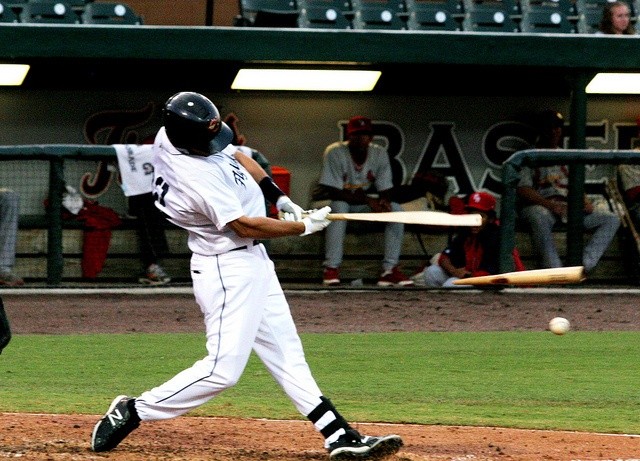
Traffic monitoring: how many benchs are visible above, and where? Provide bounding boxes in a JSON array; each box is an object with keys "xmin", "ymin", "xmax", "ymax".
[
  {"xmin": 0, "ymin": 209, "xmax": 190, "ymax": 281},
  {"xmin": 191, "ymin": 210, "xmax": 640, "ymax": 280}
]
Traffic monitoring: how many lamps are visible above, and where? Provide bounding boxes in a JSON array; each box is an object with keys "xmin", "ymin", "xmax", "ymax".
[
  {"xmin": 0, "ymin": 62, "xmax": 30, "ymax": 89},
  {"xmin": 583, "ymin": 72, "xmax": 640, "ymax": 96},
  {"xmin": 228, "ymin": 68, "xmax": 387, "ymax": 96}
]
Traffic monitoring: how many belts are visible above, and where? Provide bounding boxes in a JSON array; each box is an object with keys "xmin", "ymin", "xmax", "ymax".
[{"xmin": 230, "ymin": 239, "xmax": 261, "ymax": 252}]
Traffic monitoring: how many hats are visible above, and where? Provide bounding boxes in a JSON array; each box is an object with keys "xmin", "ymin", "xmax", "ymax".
[
  {"xmin": 538, "ymin": 110, "xmax": 563, "ymax": 125},
  {"xmin": 464, "ymin": 192, "xmax": 496, "ymax": 211},
  {"xmin": 346, "ymin": 116, "xmax": 371, "ymax": 135}
]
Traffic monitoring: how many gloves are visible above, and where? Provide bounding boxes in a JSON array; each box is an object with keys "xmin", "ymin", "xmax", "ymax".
[
  {"xmin": 301, "ymin": 206, "xmax": 331, "ymax": 237},
  {"xmin": 275, "ymin": 195, "xmax": 302, "ymax": 222}
]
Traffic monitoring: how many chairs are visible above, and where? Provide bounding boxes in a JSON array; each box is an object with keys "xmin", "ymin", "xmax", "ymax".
[
  {"xmin": 232, "ymin": 1, "xmax": 639, "ymax": 35},
  {"xmin": 1, "ymin": 2, "xmax": 147, "ymax": 27}
]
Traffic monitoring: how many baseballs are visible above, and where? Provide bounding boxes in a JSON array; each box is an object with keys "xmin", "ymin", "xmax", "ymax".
[{"xmin": 549, "ymin": 316, "xmax": 571, "ymax": 334}]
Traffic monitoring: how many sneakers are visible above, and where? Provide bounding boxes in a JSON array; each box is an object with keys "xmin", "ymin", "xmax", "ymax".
[
  {"xmin": 322, "ymin": 268, "xmax": 341, "ymax": 284},
  {"xmin": 377, "ymin": 267, "xmax": 414, "ymax": 286},
  {"xmin": 325, "ymin": 427, "xmax": 403, "ymax": 461},
  {"xmin": 139, "ymin": 266, "xmax": 171, "ymax": 286},
  {"xmin": 92, "ymin": 394, "xmax": 139, "ymax": 452}
]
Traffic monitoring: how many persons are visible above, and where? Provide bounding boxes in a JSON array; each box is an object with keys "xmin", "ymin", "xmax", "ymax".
[
  {"xmin": 425, "ymin": 195, "xmax": 525, "ymax": 289},
  {"xmin": 311, "ymin": 116, "xmax": 414, "ymax": 288},
  {"xmin": 0, "ymin": 190, "xmax": 25, "ymax": 290},
  {"xmin": 517, "ymin": 112, "xmax": 618, "ymax": 287},
  {"xmin": 593, "ymin": 2, "xmax": 638, "ymax": 38},
  {"xmin": 129, "ymin": 188, "xmax": 173, "ymax": 282},
  {"xmin": 91, "ymin": 92, "xmax": 403, "ymax": 460}
]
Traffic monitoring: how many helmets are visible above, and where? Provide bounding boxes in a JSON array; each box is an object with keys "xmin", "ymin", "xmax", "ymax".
[{"xmin": 162, "ymin": 92, "xmax": 234, "ymax": 157}]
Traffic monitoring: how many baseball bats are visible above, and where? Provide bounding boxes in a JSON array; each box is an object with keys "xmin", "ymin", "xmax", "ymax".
[
  {"xmin": 279, "ymin": 210, "xmax": 482, "ymax": 226},
  {"xmin": 453, "ymin": 266, "xmax": 588, "ymax": 286}
]
